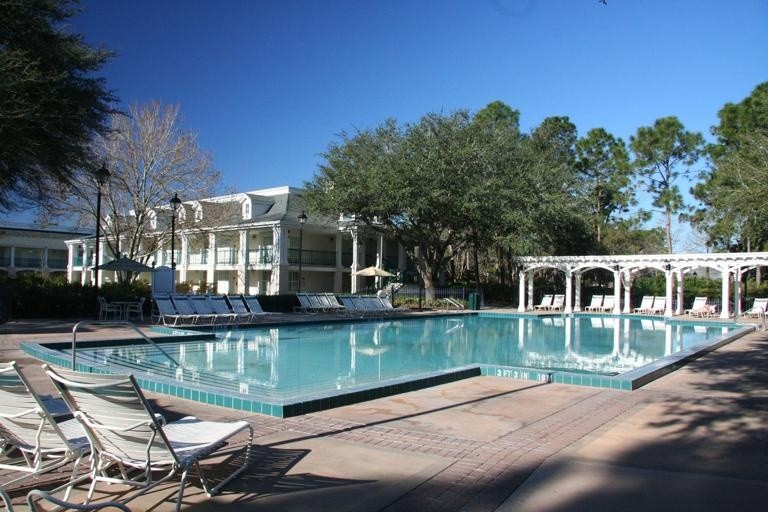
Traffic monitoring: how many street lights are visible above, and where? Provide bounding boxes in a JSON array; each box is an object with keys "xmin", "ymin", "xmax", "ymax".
[
  {"xmin": 95, "ymin": 163, "xmax": 111, "ymax": 288},
  {"xmin": 297, "ymin": 210, "xmax": 308, "ymax": 292},
  {"xmin": 169, "ymin": 194, "xmax": 181, "ymax": 270}
]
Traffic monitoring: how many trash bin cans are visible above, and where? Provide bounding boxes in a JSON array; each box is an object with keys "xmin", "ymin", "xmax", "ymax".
[{"xmin": 469, "ymin": 292, "xmax": 480, "ymax": 309}]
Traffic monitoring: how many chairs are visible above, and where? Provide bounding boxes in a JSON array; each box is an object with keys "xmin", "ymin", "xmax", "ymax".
[
  {"xmin": 0, "ymin": 359, "xmax": 255, "ymax": 512},
  {"xmin": 97, "ymin": 291, "xmax": 407, "ymax": 331},
  {"xmin": 532, "ymin": 294, "xmax": 768, "ymax": 319}
]
